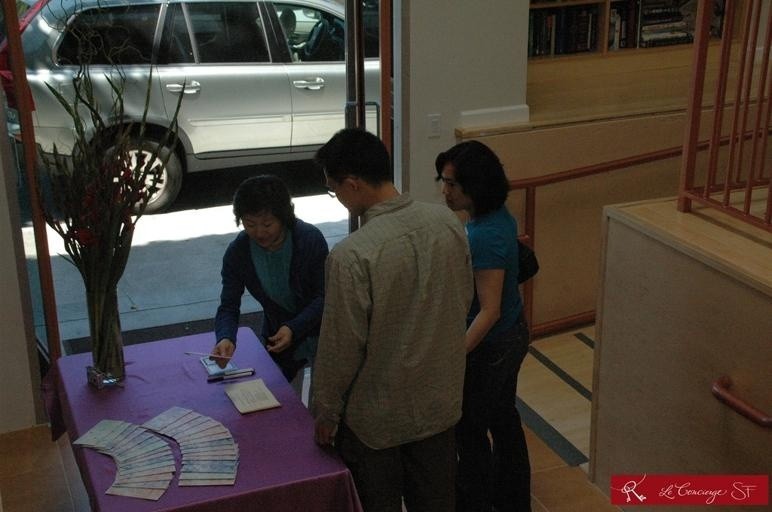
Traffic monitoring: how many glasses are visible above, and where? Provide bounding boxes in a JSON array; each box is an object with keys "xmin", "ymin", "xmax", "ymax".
[{"xmin": 327, "ymin": 176, "xmax": 359, "ymax": 198}]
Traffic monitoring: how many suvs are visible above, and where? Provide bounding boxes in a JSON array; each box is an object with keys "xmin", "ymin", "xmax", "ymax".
[{"xmin": 0, "ymin": 1, "xmax": 382, "ymax": 216}]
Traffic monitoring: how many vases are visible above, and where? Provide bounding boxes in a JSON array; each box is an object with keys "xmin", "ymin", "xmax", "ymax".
[{"xmin": 87, "ymin": 269, "xmax": 123, "ymax": 383}]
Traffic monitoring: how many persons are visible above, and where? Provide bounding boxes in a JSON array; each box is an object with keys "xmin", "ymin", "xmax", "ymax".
[
  {"xmin": 431, "ymin": 135, "xmax": 534, "ymax": 510},
  {"xmin": 207, "ymin": 173, "xmax": 330, "ymax": 387},
  {"xmin": 308, "ymin": 125, "xmax": 476, "ymax": 509}
]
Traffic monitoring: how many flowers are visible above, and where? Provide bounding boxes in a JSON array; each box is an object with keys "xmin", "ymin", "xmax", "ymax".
[{"xmin": 32, "ymin": 0, "xmax": 187, "ymax": 385}]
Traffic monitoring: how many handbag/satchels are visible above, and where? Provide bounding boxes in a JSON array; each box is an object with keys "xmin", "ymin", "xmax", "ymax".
[{"xmin": 517, "ymin": 240, "xmax": 540, "ymax": 284}]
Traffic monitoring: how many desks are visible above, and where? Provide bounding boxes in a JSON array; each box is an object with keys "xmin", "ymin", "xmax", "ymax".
[{"xmin": 47, "ymin": 328, "xmax": 351, "ymax": 510}]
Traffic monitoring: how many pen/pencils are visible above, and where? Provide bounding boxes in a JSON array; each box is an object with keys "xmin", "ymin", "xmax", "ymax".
[
  {"xmin": 208, "ymin": 367, "xmax": 255, "ymax": 378},
  {"xmin": 207, "ymin": 371, "xmax": 254, "ymax": 384},
  {"xmin": 184, "ymin": 352, "xmax": 233, "ymax": 359}
]
